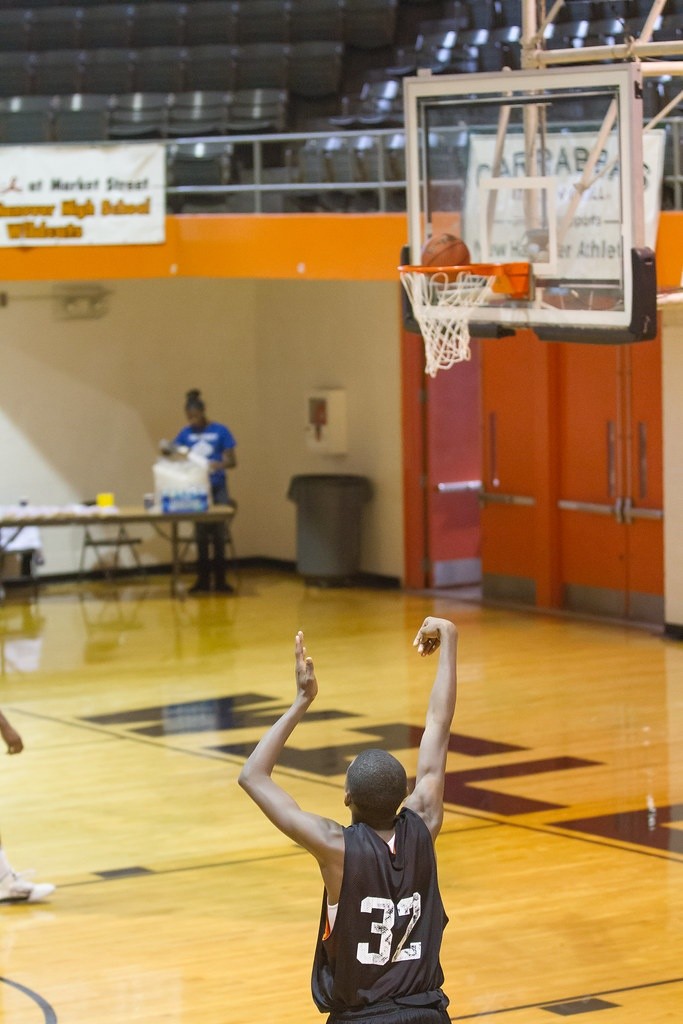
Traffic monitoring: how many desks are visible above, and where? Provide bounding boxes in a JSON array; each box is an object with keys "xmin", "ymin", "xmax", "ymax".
[{"xmin": 0, "ymin": 503, "xmax": 241, "ymax": 601}]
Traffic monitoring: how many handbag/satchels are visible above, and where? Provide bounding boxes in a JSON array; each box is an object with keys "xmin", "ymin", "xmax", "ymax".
[{"xmin": 153, "ymin": 451, "xmax": 214, "ymax": 513}]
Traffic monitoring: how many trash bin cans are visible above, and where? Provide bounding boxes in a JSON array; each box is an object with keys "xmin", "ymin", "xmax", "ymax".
[{"xmin": 288, "ymin": 475, "xmax": 375, "ymax": 580}]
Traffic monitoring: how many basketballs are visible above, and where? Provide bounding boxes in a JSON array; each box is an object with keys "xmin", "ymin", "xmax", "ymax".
[{"xmin": 422, "ymin": 233, "xmax": 471, "ymax": 284}]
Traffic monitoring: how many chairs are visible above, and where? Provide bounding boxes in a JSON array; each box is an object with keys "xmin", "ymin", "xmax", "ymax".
[
  {"xmin": 0, "ymin": 0, "xmax": 683, "ymax": 208},
  {"xmin": 76, "ymin": 500, "xmax": 145, "ymax": 589}
]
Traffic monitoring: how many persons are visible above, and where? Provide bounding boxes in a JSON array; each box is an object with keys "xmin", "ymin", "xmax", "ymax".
[
  {"xmin": 0, "ymin": 710, "xmax": 56, "ymax": 903},
  {"xmin": 159, "ymin": 389, "xmax": 237, "ymax": 596},
  {"xmin": 237, "ymin": 615, "xmax": 457, "ymax": 1024}
]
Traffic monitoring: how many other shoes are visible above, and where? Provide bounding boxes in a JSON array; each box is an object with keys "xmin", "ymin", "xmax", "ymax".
[
  {"xmin": 0, "ymin": 870, "xmax": 55, "ymax": 902},
  {"xmin": 188, "ymin": 575, "xmax": 211, "ymax": 593},
  {"xmin": 211, "ymin": 579, "xmax": 234, "ymax": 595}
]
